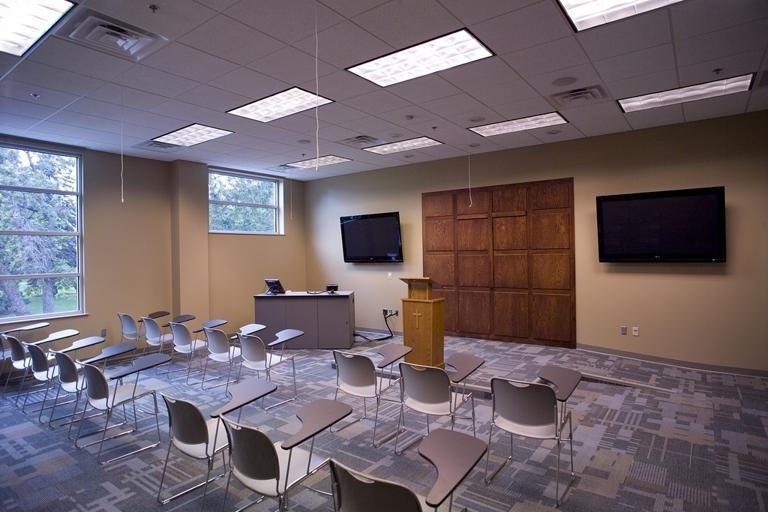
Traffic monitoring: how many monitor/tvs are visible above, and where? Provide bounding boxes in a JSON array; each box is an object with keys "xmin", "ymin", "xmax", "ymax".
[
  {"xmin": 340, "ymin": 211, "xmax": 404, "ymax": 263},
  {"xmin": 596, "ymin": 185, "xmax": 726, "ymax": 263}
]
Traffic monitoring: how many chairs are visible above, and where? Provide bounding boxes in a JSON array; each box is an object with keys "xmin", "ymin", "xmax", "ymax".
[
  {"xmin": 0, "ymin": 306, "xmax": 303, "ymax": 465},
  {"xmin": 157, "ymin": 342, "xmax": 582, "ymax": 511}
]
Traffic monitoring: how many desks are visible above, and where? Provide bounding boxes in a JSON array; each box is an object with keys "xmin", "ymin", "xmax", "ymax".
[{"xmin": 254, "ymin": 289, "xmax": 356, "ymax": 353}]
